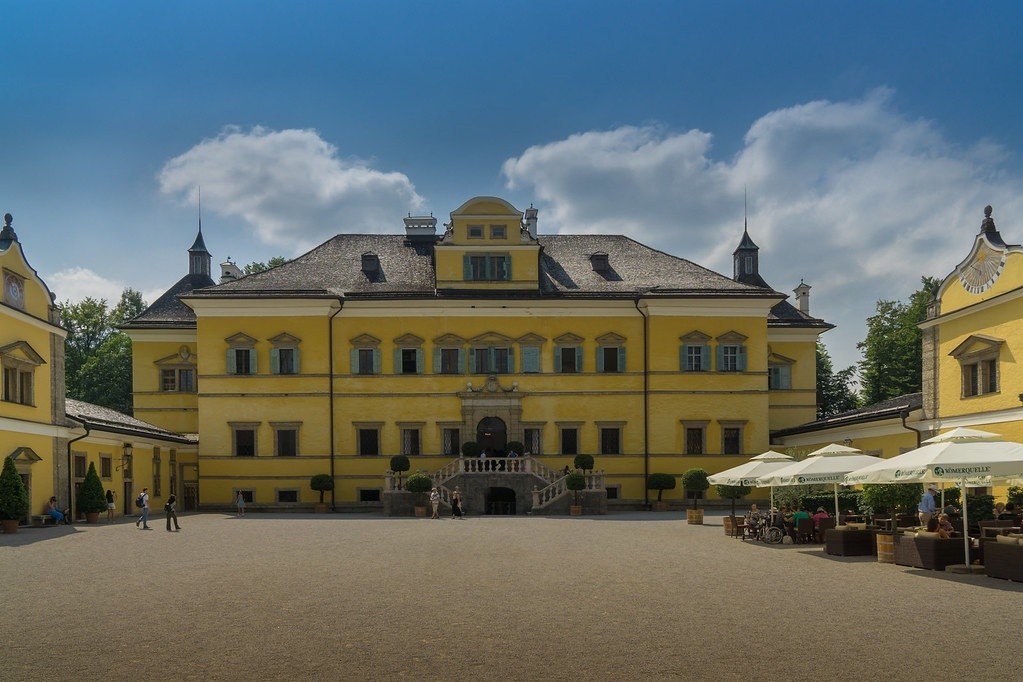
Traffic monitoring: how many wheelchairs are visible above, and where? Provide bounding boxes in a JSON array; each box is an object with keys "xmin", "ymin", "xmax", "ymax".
[{"xmin": 758, "ymin": 518, "xmax": 797, "ymax": 545}]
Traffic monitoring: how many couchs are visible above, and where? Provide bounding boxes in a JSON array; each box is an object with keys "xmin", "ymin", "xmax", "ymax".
[
  {"xmin": 893, "ymin": 533, "xmax": 1023, "ymax": 582},
  {"xmin": 825, "ymin": 525, "xmax": 883, "ymax": 556}
]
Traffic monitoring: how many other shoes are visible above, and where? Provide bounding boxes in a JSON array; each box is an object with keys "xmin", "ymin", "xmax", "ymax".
[
  {"xmin": 176, "ymin": 527, "xmax": 181, "ymax": 529},
  {"xmin": 144, "ymin": 526, "xmax": 150, "ymax": 529},
  {"xmin": 136, "ymin": 521, "xmax": 140, "ymax": 527}
]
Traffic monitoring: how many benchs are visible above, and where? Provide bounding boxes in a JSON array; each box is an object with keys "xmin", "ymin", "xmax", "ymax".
[{"xmin": 32, "ymin": 514, "xmax": 69, "ymax": 528}]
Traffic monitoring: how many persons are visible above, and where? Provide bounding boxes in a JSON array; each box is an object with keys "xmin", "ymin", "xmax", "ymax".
[
  {"xmin": 237, "ymin": 491, "xmax": 245, "ymax": 516},
  {"xmin": 452, "ymin": 486, "xmax": 463, "ymax": 519},
  {"xmin": 936, "ymin": 513, "xmax": 953, "ymax": 533},
  {"xmin": 430, "ymin": 488, "xmax": 440, "ymax": 519},
  {"xmin": 166, "ymin": 495, "xmax": 181, "ymax": 530},
  {"xmin": 813, "ymin": 507, "xmax": 828, "ymax": 543},
  {"xmin": 790, "ymin": 506, "xmax": 809, "ymax": 543},
  {"xmin": 47, "ymin": 497, "xmax": 69, "ymax": 526},
  {"xmin": 998, "ymin": 502, "xmax": 1018, "ymax": 520},
  {"xmin": 747, "ymin": 504, "xmax": 761, "ymax": 537},
  {"xmin": 918, "ymin": 485, "xmax": 938, "ymax": 527},
  {"xmin": 480, "ymin": 450, "xmax": 486, "ymax": 471},
  {"xmin": 136, "ymin": 488, "xmax": 150, "ymax": 528},
  {"xmin": 776, "ymin": 507, "xmax": 790, "ymax": 536},
  {"xmin": 790, "ymin": 506, "xmax": 798, "ymax": 517},
  {"xmin": 993, "ymin": 503, "xmax": 1005, "ymax": 519},
  {"xmin": 564, "ymin": 466, "xmax": 570, "ymax": 475},
  {"xmin": 507, "ymin": 450, "xmax": 518, "ymax": 458},
  {"xmin": 106, "ymin": 490, "xmax": 116, "ymax": 524}
]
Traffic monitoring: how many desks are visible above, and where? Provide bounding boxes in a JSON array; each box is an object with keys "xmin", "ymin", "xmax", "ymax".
[
  {"xmin": 874, "ymin": 518, "xmax": 901, "ymax": 530},
  {"xmin": 846, "ymin": 515, "xmax": 868, "ymax": 524}
]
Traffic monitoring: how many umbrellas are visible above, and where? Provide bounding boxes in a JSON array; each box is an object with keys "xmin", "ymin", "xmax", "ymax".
[{"xmin": 706, "ymin": 427, "xmax": 1023, "ymax": 566}]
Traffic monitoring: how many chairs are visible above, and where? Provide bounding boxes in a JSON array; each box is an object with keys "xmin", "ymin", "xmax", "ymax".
[
  {"xmin": 901, "ymin": 516, "xmax": 1023, "ymax": 537},
  {"xmin": 729, "ymin": 514, "xmax": 836, "ymax": 544}
]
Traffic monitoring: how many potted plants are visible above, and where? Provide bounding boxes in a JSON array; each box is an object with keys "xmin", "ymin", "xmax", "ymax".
[
  {"xmin": 405, "ymin": 472, "xmax": 433, "ymax": 517},
  {"xmin": 863, "ymin": 482, "xmax": 925, "ymax": 564},
  {"xmin": 715, "ymin": 484, "xmax": 752, "ymax": 536},
  {"xmin": 77, "ymin": 460, "xmax": 108, "ymax": 525},
  {"xmin": 309, "ymin": 474, "xmax": 334, "ymax": 514},
  {"xmin": 648, "ymin": 473, "xmax": 676, "ymax": 512},
  {"xmin": 0, "ymin": 455, "xmax": 27, "ymax": 533},
  {"xmin": 682, "ymin": 469, "xmax": 710, "ymax": 524},
  {"xmin": 565, "ymin": 473, "xmax": 586, "ymax": 515}
]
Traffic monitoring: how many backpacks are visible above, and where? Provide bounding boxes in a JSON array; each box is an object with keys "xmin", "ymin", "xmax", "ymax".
[
  {"xmin": 136, "ymin": 493, "xmax": 146, "ymax": 508},
  {"xmin": 164, "ymin": 503, "xmax": 172, "ymax": 512}
]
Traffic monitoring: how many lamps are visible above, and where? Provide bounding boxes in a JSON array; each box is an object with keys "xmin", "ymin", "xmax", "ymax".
[{"xmin": 115, "ymin": 443, "xmax": 133, "ymax": 471}]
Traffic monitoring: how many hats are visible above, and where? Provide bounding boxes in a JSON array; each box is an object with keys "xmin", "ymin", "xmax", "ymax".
[
  {"xmin": 455, "ymin": 486, "xmax": 459, "ymax": 489},
  {"xmin": 928, "ymin": 485, "xmax": 939, "ymax": 493},
  {"xmin": 432, "ymin": 488, "xmax": 437, "ymax": 490},
  {"xmin": 817, "ymin": 507, "xmax": 824, "ymax": 511}
]
